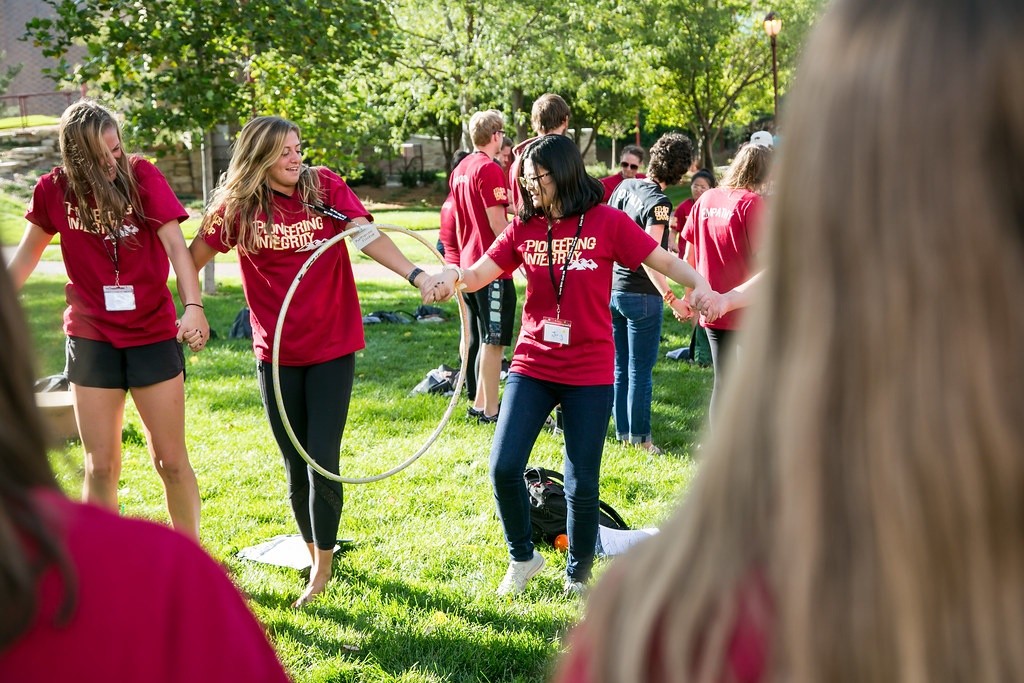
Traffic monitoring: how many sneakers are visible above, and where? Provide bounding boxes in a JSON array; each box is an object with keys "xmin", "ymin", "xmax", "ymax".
[
  {"xmin": 496, "ymin": 549, "xmax": 545, "ymax": 598},
  {"xmin": 563, "ymin": 579, "xmax": 586, "ymax": 596}
]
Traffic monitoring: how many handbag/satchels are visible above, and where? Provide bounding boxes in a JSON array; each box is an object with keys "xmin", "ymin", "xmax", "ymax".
[
  {"xmin": 230, "ymin": 304, "xmax": 252, "ymax": 338},
  {"xmin": 407, "ymin": 356, "xmax": 467, "ymax": 398},
  {"xmin": 689, "ymin": 319, "xmax": 712, "ymax": 367},
  {"xmin": 523, "ymin": 465, "xmax": 631, "ymax": 550},
  {"xmin": 361, "ymin": 303, "xmax": 453, "ymax": 324}
]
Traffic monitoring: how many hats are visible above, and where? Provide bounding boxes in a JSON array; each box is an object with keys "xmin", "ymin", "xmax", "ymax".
[{"xmin": 750, "ymin": 130, "xmax": 773, "ymax": 147}]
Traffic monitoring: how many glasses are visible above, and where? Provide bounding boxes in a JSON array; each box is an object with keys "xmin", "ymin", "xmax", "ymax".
[
  {"xmin": 493, "ymin": 129, "xmax": 504, "ymax": 136},
  {"xmin": 621, "ymin": 158, "xmax": 640, "ymax": 171},
  {"xmin": 517, "ymin": 172, "xmax": 553, "ymax": 190}
]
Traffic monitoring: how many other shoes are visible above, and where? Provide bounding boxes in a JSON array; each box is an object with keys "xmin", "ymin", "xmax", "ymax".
[
  {"xmin": 553, "ymin": 427, "xmax": 564, "ymax": 436},
  {"xmin": 476, "ymin": 409, "xmax": 498, "ymax": 425},
  {"xmin": 465, "ymin": 405, "xmax": 483, "ymax": 421},
  {"xmin": 543, "ymin": 415, "xmax": 556, "ymax": 429}
]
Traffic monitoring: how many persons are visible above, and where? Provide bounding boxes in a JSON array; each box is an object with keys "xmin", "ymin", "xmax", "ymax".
[
  {"xmin": 559, "ymin": 0, "xmax": 1024, "ymax": 683},
  {"xmin": 421, "ymin": 134, "xmax": 723, "ymax": 606},
  {"xmin": 598, "ymin": 146, "xmax": 647, "ymax": 203},
  {"xmin": 437, "ymin": 108, "xmax": 517, "ymax": 425},
  {"xmin": 670, "ymin": 144, "xmax": 777, "ymax": 431},
  {"xmin": 606, "ymin": 133, "xmax": 694, "ymax": 453},
  {"xmin": 508, "ymin": 93, "xmax": 572, "ymax": 435},
  {"xmin": 177, "ymin": 116, "xmax": 450, "ymax": 607},
  {"xmin": 0, "ymin": 257, "xmax": 290, "ymax": 683},
  {"xmin": 10, "ymin": 99, "xmax": 210, "ymax": 542}
]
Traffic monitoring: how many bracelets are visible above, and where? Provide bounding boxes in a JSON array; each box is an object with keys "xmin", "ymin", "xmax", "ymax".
[
  {"xmin": 409, "ymin": 268, "xmax": 424, "ymax": 288},
  {"xmin": 663, "ymin": 290, "xmax": 675, "ymax": 304},
  {"xmin": 185, "ymin": 303, "xmax": 204, "ymax": 309}
]
